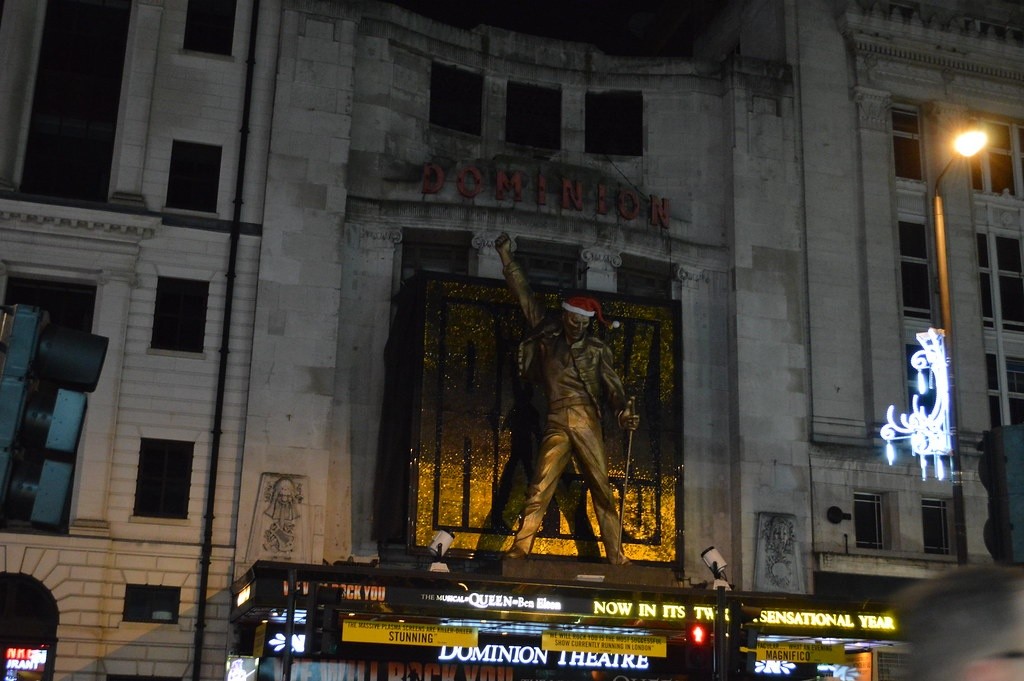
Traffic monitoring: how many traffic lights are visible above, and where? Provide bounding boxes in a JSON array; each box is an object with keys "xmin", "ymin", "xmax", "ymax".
[
  {"xmin": 739, "ymin": 606, "xmax": 764, "ymax": 678},
  {"xmin": 0, "ymin": 321, "xmax": 108, "ymax": 535},
  {"xmin": 975, "ymin": 425, "xmax": 1007, "ymax": 564},
  {"xmin": 689, "ymin": 623, "xmax": 706, "ymax": 670}
]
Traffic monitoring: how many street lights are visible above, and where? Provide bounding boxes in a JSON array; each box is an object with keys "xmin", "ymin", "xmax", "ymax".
[{"xmin": 933, "ymin": 125, "xmax": 993, "ymax": 565}]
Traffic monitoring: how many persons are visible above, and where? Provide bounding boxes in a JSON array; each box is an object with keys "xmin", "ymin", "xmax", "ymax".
[{"xmin": 495, "ymin": 232, "xmax": 640, "ymax": 565}]
